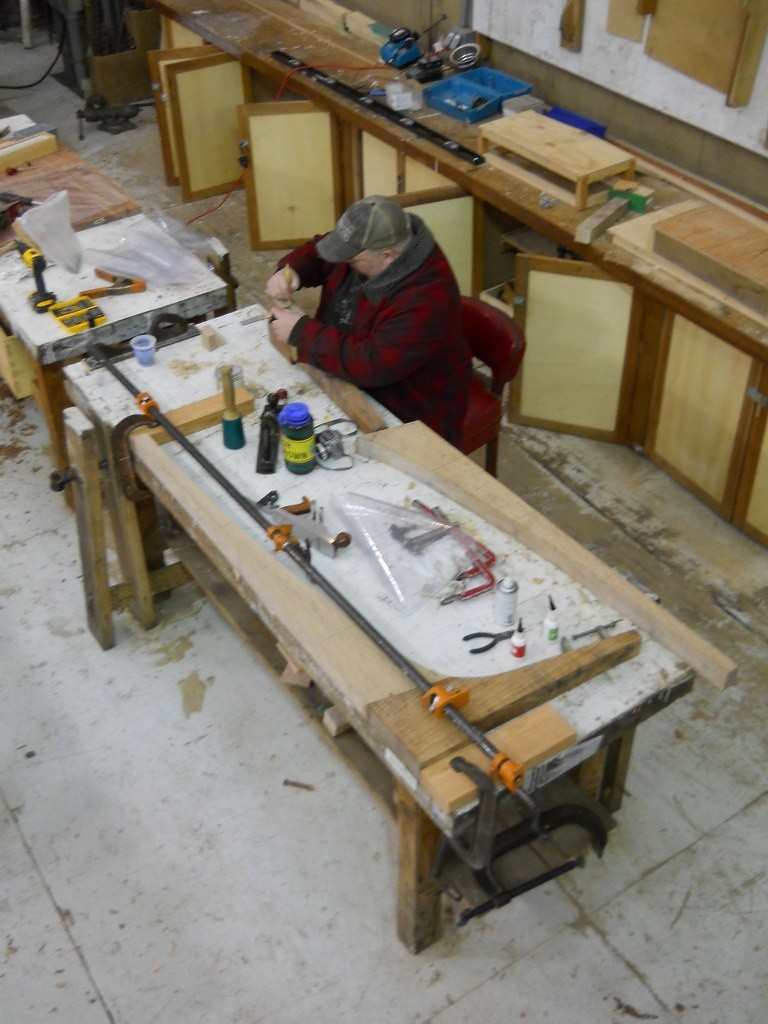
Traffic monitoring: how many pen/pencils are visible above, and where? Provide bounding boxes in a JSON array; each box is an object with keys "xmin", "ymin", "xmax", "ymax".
[{"xmin": 285, "ymin": 263, "xmax": 292, "ymax": 306}]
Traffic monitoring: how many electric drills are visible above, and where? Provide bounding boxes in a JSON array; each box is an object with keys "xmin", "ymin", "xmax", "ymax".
[{"xmin": 13, "ymin": 238, "xmax": 57, "ymax": 314}]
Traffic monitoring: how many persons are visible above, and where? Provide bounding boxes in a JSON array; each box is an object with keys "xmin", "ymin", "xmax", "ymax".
[{"xmin": 264, "ymin": 194, "xmax": 474, "ymax": 447}]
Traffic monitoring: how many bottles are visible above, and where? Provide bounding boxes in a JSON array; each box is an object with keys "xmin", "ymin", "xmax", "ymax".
[
  {"xmin": 510, "ymin": 630, "xmax": 526, "ymax": 659},
  {"xmin": 495, "ymin": 577, "xmax": 519, "ymax": 627},
  {"xmin": 278, "ymin": 401, "xmax": 316, "ymax": 475},
  {"xmin": 543, "ymin": 608, "xmax": 561, "ymax": 643}
]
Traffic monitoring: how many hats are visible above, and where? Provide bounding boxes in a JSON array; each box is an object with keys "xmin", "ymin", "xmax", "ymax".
[{"xmin": 317, "ymin": 194, "xmax": 407, "ymax": 263}]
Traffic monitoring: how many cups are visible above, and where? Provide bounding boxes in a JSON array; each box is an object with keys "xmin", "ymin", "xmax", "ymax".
[
  {"xmin": 130, "ymin": 334, "xmax": 157, "ymax": 366},
  {"xmin": 215, "ymin": 364, "xmax": 244, "ymax": 393}
]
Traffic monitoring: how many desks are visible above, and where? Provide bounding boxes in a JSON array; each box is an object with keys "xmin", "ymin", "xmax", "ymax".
[
  {"xmin": 0, "ymin": 101, "xmax": 236, "ymax": 506},
  {"xmin": 61, "ymin": 302, "xmax": 698, "ymax": 956}
]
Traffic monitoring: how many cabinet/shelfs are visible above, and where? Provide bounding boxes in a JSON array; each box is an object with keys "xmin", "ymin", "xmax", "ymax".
[{"xmin": 146, "ymin": 0, "xmax": 768, "ymax": 548}]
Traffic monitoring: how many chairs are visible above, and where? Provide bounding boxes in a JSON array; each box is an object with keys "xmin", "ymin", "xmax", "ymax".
[{"xmin": 452, "ymin": 296, "xmax": 526, "ymax": 481}]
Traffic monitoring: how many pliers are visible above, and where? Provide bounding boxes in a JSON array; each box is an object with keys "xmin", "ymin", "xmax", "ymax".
[
  {"xmin": 80, "ymin": 268, "xmax": 147, "ymax": 299},
  {"xmin": 462, "ymin": 628, "xmax": 526, "ymax": 654}
]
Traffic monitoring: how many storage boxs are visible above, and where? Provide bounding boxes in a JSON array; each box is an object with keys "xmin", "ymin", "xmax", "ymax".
[{"xmin": 0, "ymin": 323, "xmax": 83, "ymax": 399}]
